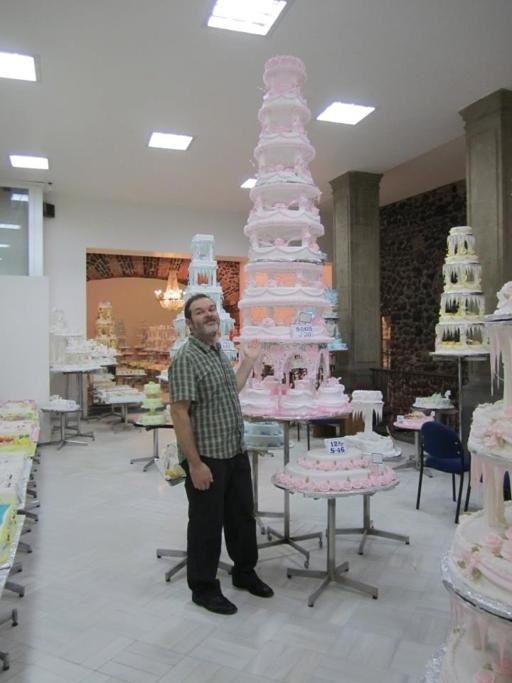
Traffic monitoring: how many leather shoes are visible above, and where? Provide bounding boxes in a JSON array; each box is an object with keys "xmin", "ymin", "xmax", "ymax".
[
  {"xmin": 191, "ymin": 579, "xmax": 237, "ymax": 614},
  {"xmin": 231, "ymin": 567, "xmax": 274, "ymax": 597}
]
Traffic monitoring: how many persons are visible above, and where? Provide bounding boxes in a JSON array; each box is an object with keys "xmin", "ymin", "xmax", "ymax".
[{"xmin": 169, "ymin": 295, "xmax": 273, "ymax": 612}]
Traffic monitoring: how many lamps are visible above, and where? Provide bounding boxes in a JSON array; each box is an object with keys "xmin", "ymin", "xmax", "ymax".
[{"xmin": 154, "ymin": 270, "xmax": 186, "ymax": 311}]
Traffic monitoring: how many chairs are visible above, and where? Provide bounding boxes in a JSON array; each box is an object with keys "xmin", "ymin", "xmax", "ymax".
[{"xmin": 415, "ymin": 420, "xmax": 473, "ymax": 523}]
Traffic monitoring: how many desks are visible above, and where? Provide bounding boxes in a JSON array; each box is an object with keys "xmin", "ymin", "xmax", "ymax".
[
  {"xmin": 40, "ymin": 358, "xmax": 144, "ymax": 450},
  {"xmin": 0, "ymin": 399, "xmax": 39, "ymax": 671},
  {"xmin": 394, "ymin": 356, "xmax": 490, "ymax": 477}
]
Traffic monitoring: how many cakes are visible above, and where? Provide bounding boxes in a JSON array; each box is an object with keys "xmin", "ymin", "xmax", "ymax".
[
  {"xmin": 350, "ymin": 386, "xmax": 386, "ymax": 408},
  {"xmin": 138, "ymin": 381, "xmax": 166, "ymax": 426},
  {"xmin": 345, "ymin": 431, "xmax": 396, "ymax": 456},
  {"xmin": 170, "ymin": 230, "xmax": 237, "ymax": 363},
  {"xmin": 426, "ymin": 280, "xmax": 512, "ymax": 683},
  {"xmin": 275, "ymin": 445, "xmax": 399, "ymax": 491},
  {"xmin": 50, "ymin": 397, "xmax": 80, "ymax": 411},
  {"xmin": 430, "ymin": 224, "xmax": 487, "ymax": 350},
  {"xmin": 235, "ymin": 51, "xmax": 348, "ymax": 421},
  {"xmin": 411, "ymin": 394, "xmax": 453, "ymax": 411}
]
